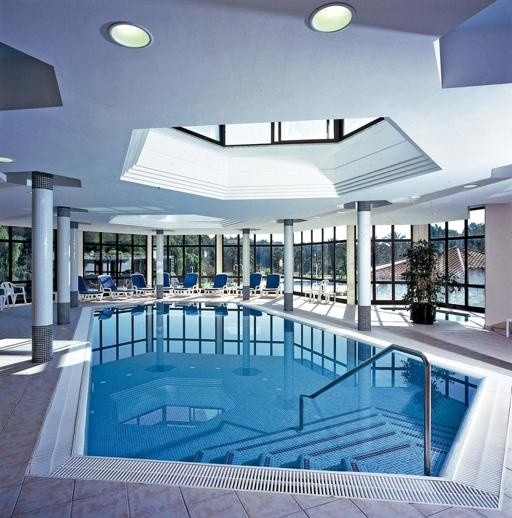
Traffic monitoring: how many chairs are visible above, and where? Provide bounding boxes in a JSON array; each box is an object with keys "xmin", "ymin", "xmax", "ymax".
[
  {"xmin": 164, "ymin": 273, "xmax": 173, "ymax": 297},
  {"xmin": 0, "ymin": 281, "xmax": 27, "ymax": 312},
  {"xmin": 204, "ymin": 274, "xmax": 228, "ymax": 298},
  {"xmin": 215, "ymin": 304, "xmax": 228, "ymax": 316},
  {"xmin": 131, "ymin": 275, "xmax": 156, "ymax": 297},
  {"xmin": 163, "ymin": 304, "xmax": 169, "ymax": 314},
  {"xmin": 78, "ymin": 276, "xmax": 104, "ymax": 302},
  {"xmin": 186, "ymin": 303, "xmax": 198, "ymax": 315},
  {"xmin": 236, "ymin": 274, "xmax": 261, "ymax": 298},
  {"xmin": 261, "ymin": 275, "xmax": 282, "ymax": 298},
  {"xmin": 131, "ymin": 305, "xmax": 145, "ymax": 316},
  {"xmin": 250, "ymin": 308, "xmax": 262, "ymax": 316},
  {"xmin": 98, "ymin": 275, "xmax": 134, "ymax": 300},
  {"xmin": 98, "ymin": 308, "xmax": 115, "ymax": 319},
  {"xmin": 304, "ymin": 280, "xmax": 336, "ymax": 304},
  {"xmin": 174, "ymin": 274, "xmax": 199, "ymax": 297}
]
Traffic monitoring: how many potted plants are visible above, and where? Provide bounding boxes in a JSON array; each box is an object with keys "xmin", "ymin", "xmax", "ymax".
[{"xmin": 401, "ymin": 239, "xmax": 465, "ymax": 325}]
